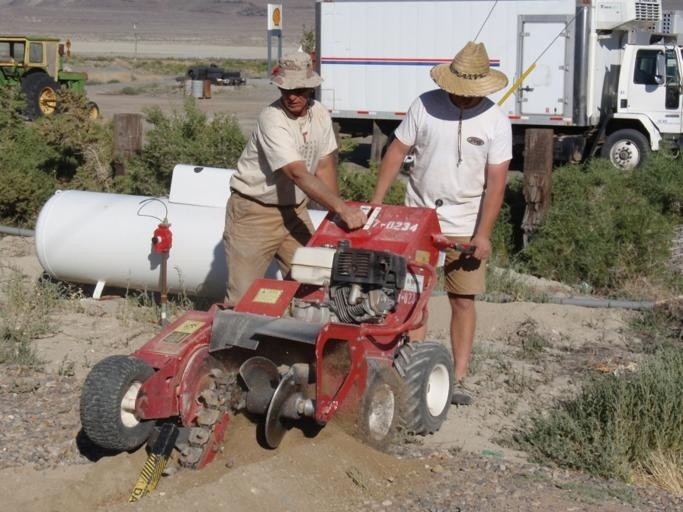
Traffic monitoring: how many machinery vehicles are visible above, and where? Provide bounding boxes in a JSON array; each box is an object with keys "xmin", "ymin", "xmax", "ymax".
[
  {"xmin": 0, "ymin": 33, "xmax": 100, "ymax": 125},
  {"xmin": 78, "ymin": 191, "xmax": 454, "ymax": 504}
]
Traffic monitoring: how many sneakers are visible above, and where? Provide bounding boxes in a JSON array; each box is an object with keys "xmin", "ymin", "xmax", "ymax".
[{"xmin": 446, "ymin": 373, "xmax": 472, "ymax": 407}]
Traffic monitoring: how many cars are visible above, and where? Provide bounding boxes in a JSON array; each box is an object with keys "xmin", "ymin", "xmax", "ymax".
[{"xmin": 189, "ymin": 63, "xmax": 248, "ymax": 86}]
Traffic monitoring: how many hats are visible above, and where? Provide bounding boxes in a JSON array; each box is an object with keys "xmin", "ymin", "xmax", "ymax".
[
  {"xmin": 428, "ymin": 42, "xmax": 510, "ymax": 100},
  {"xmin": 270, "ymin": 45, "xmax": 325, "ymax": 92}
]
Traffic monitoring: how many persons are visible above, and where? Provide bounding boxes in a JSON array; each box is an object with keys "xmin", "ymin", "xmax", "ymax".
[
  {"xmin": 365, "ymin": 40, "xmax": 516, "ymax": 405},
  {"xmin": 222, "ymin": 51, "xmax": 367, "ymax": 308}
]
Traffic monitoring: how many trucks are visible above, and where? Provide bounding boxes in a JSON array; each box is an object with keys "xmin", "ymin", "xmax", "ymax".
[{"xmin": 311, "ymin": 1, "xmax": 682, "ymax": 177}]
